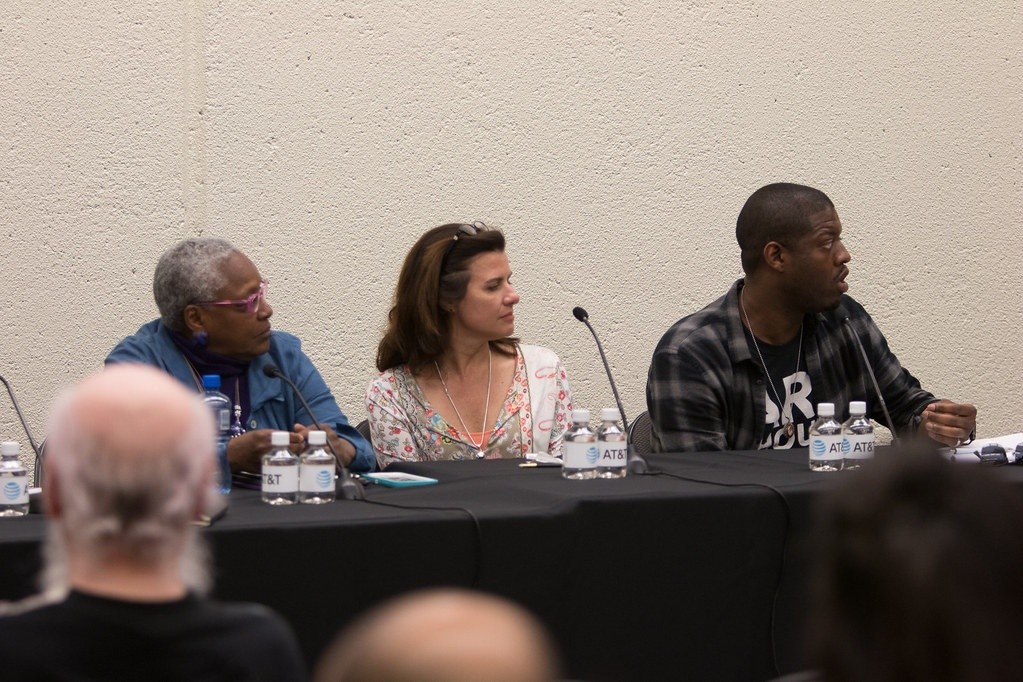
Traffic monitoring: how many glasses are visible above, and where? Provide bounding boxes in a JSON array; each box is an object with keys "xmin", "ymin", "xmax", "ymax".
[
  {"xmin": 195, "ymin": 279, "xmax": 268, "ymax": 313},
  {"xmin": 443, "ymin": 221, "xmax": 489, "ymax": 259}
]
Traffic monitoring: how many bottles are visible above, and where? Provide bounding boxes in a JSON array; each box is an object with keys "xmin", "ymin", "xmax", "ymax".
[
  {"xmin": 261, "ymin": 432, "xmax": 300, "ymax": 504},
  {"xmin": 595, "ymin": 408, "xmax": 628, "ymax": 478},
  {"xmin": 562, "ymin": 410, "xmax": 598, "ymax": 479},
  {"xmin": 809, "ymin": 403, "xmax": 843, "ymax": 470},
  {"xmin": 842, "ymin": 402, "xmax": 874, "ymax": 470},
  {"xmin": 298, "ymin": 430, "xmax": 336, "ymax": 504},
  {"xmin": 0, "ymin": 442, "xmax": 31, "ymax": 517},
  {"xmin": 196, "ymin": 375, "xmax": 232, "ymax": 495}
]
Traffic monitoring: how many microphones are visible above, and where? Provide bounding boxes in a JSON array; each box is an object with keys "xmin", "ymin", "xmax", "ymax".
[
  {"xmin": 573, "ymin": 306, "xmax": 649, "ymax": 475},
  {"xmin": 262, "ymin": 363, "xmax": 366, "ymax": 501},
  {"xmin": 833, "ymin": 306, "xmax": 903, "ymax": 448}
]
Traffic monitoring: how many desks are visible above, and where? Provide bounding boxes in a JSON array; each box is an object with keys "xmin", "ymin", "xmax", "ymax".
[{"xmin": 0, "ymin": 450, "xmax": 1023, "ymax": 682}]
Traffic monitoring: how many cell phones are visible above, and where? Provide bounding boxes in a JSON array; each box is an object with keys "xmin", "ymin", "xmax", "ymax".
[{"xmin": 362, "ymin": 471, "xmax": 439, "ymax": 488}]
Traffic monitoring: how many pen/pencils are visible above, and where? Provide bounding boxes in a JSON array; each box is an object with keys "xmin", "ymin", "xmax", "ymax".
[{"xmin": 519, "ymin": 462, "xmax": 563, "ymax": 467}]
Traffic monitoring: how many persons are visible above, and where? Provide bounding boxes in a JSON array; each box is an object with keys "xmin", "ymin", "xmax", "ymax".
[
  {"xmin": 318, "ymin": 587, "xmax": 557, "ymax": 682},
  {"xmin": 363, "ymin": 224, "xmax": 573, "ymax": 470},
  {"xmin": 0, "ymin": 361, "xmax": 300, "ymax": 682},
  {"xmin": 646, "ymin": 183, "xmax": 977, "ymax": 455},
  {"xmin": 799, "ymin": 436, "xmax": 1023, "ymax": 682},
  {"xmin": 103, "ymin": 236, "xmax": 377, "ymax": 483}
]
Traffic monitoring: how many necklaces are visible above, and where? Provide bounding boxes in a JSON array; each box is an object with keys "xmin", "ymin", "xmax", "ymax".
[
  {"xmin": 186, "ymin": 356, "xmax": 246, "ymax": 439},
  {"xmin": 742, "ymin": 285, "xmax": 803, "ymax": 438},
  {"xmin": 434, "ymin": 350, "xmax": 491, "ymax": 459}
]
